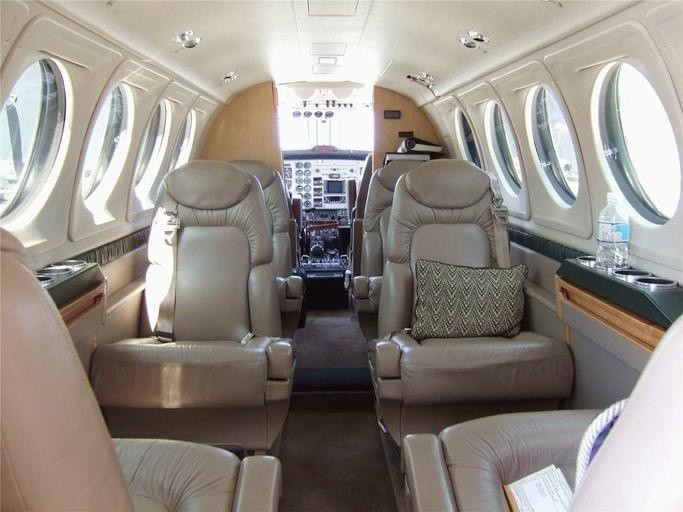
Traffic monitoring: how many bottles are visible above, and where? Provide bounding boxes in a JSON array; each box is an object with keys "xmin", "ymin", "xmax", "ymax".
[{"xmin": 594, "ymin": 191, "xmax": 632, "ymax": 274}]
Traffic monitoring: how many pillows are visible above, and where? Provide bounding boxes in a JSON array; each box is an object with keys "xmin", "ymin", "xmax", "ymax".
[{"xmin": 409, "ymin": 255, "xmax": 534, "ymax": 344}]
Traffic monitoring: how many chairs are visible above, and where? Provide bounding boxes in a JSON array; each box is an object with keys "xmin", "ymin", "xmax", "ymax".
[
  {"xmin": 1, "ymin": 226, "xmax": 283, "ymax": 512},
  {"xmin": 401, "ymin": 310, "xmax": 683, "ymax": 512},
  {"xmin": 347, "ymin": 148, "xmax": 422, "ymax": 319},
  {"xmin": 84, "ymin": 156, "xmax": 309, "ymax": 454},
  {"xmin": 365, "ymin": 156, "xmax": 579, "ymax": 437}
]
